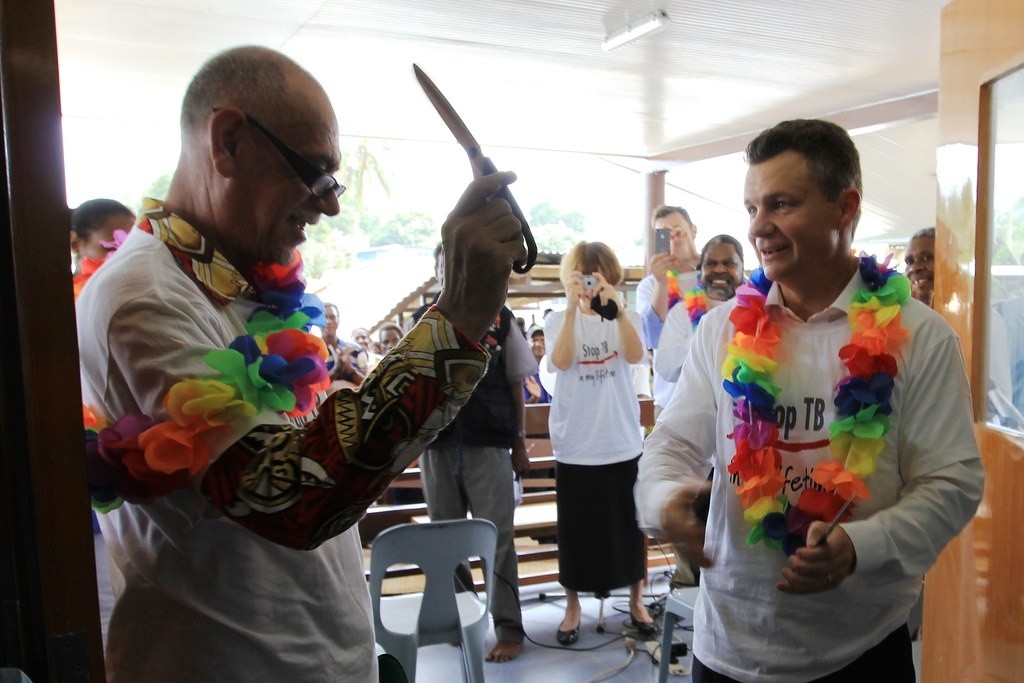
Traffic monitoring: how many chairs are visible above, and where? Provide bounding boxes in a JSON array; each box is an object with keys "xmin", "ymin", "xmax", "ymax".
[
  {"xmin": 371, "ymin": 516, "xmax": 497, "ymax": 683},
  {"xmin": 657, "ymin": 585, "xmax": 702, "ymax": 683}
]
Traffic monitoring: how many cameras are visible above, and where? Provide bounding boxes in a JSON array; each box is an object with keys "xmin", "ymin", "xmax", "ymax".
[{"xmin": 577, "ymin": 275, "xmax": 597, "ymax": 289}]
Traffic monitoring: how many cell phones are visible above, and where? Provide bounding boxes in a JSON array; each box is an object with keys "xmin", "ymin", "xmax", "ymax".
[{"xmin": 655, "ymin": 229, "xmax": 671, "ymax": 256}]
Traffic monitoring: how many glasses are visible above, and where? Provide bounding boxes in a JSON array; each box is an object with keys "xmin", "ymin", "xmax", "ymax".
[{"xmin": 213, "ymin": 106, "xmax": 347, "ymax": 200}]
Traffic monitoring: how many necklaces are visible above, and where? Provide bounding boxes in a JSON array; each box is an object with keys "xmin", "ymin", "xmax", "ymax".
[
  {"xmin": 666, "ymin": 263, "xmax": 703, "ymax": 310},
  {"xmin": 720, "ymin": 254, "xmax": 910, "ymax": 556},
  {"xmin": 683, "ymin": 289, "xmax": 706, "ymax": 332},
  {"xmin": 73, "ymin": 227, "xmax": 337, "ymax": 516}
]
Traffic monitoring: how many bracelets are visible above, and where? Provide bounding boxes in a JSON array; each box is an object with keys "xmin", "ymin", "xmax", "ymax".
[{"xmin": 350, "ymin": 369, "xmax": 357, "ymax": 376}]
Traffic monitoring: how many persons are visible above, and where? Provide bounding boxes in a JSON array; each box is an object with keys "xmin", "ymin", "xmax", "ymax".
[
  {"xmin": 71, "ymin": 199, "xmax": 136, "ymax": 281},
  {"xmin": 654, "ymin": 235, "xmax": 745, "ymax": 384},
  {"xmin": 76, "ymin": 45, "xmax": 527, "ymax": 683},
  {"xmin": 634, "ymin": 118, "xmax": 983, "ymax": 683},
  {"xmin": 514, "ymin": 308, "xmax": 553, "ymax": 404},
  {"xmin": 402, "ymin": 241, "xmax": 538, "ymax": 663},
  {"xmin": 905, "ymin": 228, "xmax": 935, "ymax": 310},
  {"xmin": 635, "ymin": 204, "xmax": 702, "ymax": 590},
  {"xmin": 316, "ymin": 302, "xmax": 426, "ymax": 505},
  {"xmin": 540, "ymin": 242, "xmax": 660, "ymax": 644}
]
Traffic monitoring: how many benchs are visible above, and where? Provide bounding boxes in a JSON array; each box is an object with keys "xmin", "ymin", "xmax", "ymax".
[{"xmin": 410, "ymin": 456, "xmax": 675, "ymax": 592}]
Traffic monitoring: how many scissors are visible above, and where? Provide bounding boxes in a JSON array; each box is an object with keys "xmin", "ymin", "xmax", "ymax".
[
  {"xmin": 412, "ymin": 63, "xmax": 539, "ymax": 274},
  {"xmin": 814, "ymin": 494, "xmax": 856, "ymax": 545}
]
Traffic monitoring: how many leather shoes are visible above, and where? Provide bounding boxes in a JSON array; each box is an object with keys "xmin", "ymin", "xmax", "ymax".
[
  {"xmin": 556, "ymin": 607, "xmax": 581, "ymax": 645},
  {"xmin": 628, "ymin": 600, "xmax": 659, "ymax": 634}
]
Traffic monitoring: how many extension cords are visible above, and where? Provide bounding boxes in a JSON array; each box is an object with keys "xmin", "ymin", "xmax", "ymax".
[{"xmin": 646, "ymin": 640, "xmax": 684, "ymax": 675}]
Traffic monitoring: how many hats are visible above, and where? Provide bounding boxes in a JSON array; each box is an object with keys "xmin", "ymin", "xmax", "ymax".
[{"xmin": 528, "ymin": 326, "xmax": 545, "ymax": 338}]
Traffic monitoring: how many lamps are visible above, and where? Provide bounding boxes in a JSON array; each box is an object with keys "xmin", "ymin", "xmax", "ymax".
[{"xmin": 604, "ymin": 9, "xmax": 668, "ymax": 51}]
